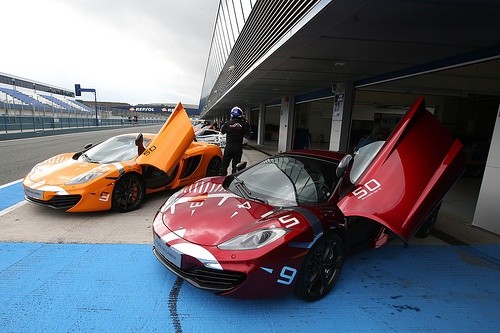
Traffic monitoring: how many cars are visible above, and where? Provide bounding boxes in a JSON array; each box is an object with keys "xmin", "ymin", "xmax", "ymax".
[{"xmin": 191, "ymin": 128, "xmax": 248, "ymax": 148}]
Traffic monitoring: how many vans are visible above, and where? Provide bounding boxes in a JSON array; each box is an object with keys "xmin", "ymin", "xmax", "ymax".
[{"xmin": 190, "ymin": 120, "xmax": 214, "ymax": 131}]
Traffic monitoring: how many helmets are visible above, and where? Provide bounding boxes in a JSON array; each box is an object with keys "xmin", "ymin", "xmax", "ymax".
[{"xmin": 231, "ymin": 107, "xmax": 243, "ymax": 119}]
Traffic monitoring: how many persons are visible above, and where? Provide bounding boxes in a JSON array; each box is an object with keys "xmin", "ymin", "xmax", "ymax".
[
  {"xmin": 221, "ymin": 106, "xmax": 250, "ymax": 177},
  {"xmin": 128, "ymin": 115, "xmax": 138, "ymax": 125}
]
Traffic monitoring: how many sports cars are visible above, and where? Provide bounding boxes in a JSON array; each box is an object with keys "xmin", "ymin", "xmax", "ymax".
[
  {"xmin": 22, "ymin": 102, "xmax": 224, "ymax": 212},
  {"xmin": 150, "ymin": 95, "xmax": 470, "ymax": 303}
]
